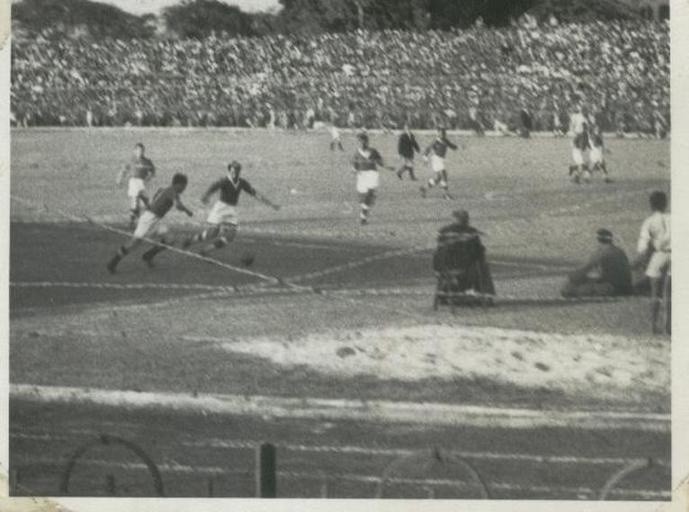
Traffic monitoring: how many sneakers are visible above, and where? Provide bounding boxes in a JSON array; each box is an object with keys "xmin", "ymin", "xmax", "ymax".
[
  {"xmin": 569, "ymin": 166, "xmax": 614, "ymax": 184},
  {"xmin": 105, "ymin": 261, "xmax": 115, "ymax": 276},
  {"xmin": 142, "ymin": 253, "xmax": 152, "ymax": 267},
  {"xmin": 419, "ymin": 185, "xmax": 455, "ymax": 200}
]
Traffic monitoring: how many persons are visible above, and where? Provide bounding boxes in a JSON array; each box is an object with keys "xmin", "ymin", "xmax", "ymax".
[
  {"xmin": 559, "ymin": 228, "xmax": 633, "ymax": 298},
  {"xmin": 586, "ymin": 125, "xmax": 609, "ymax": 183},
  {"xmin": 348, "ymin": 127, "xmax": 395, "ymax": 225},
  {"xmin": 397, "ymin": 123, "xmax": 421, "ymax": 181},
  {"xmin": 568, "ymin": 122, "xmax": 592, "ymax": 183},
  {"xmin": 107, "ymin": 172, "xmax": 193, "ymax": 274},
  {"xmin": 431, "ymin": 208, "xmax": 496, "ymax": 294},
  {"xmin": 421, "ymin": 127, "xmax": 458, "ymax": 201},
  {"xmin": 630, "ymin": 189, "xmax": 671, "ymax": 335},
  {"xmin": 117, "ymin": 143, "xmax": 156, "ymax": 227},
  {"xmin": 182, "ymin": 158, "xmax": 281, "ymax": 256}
]
